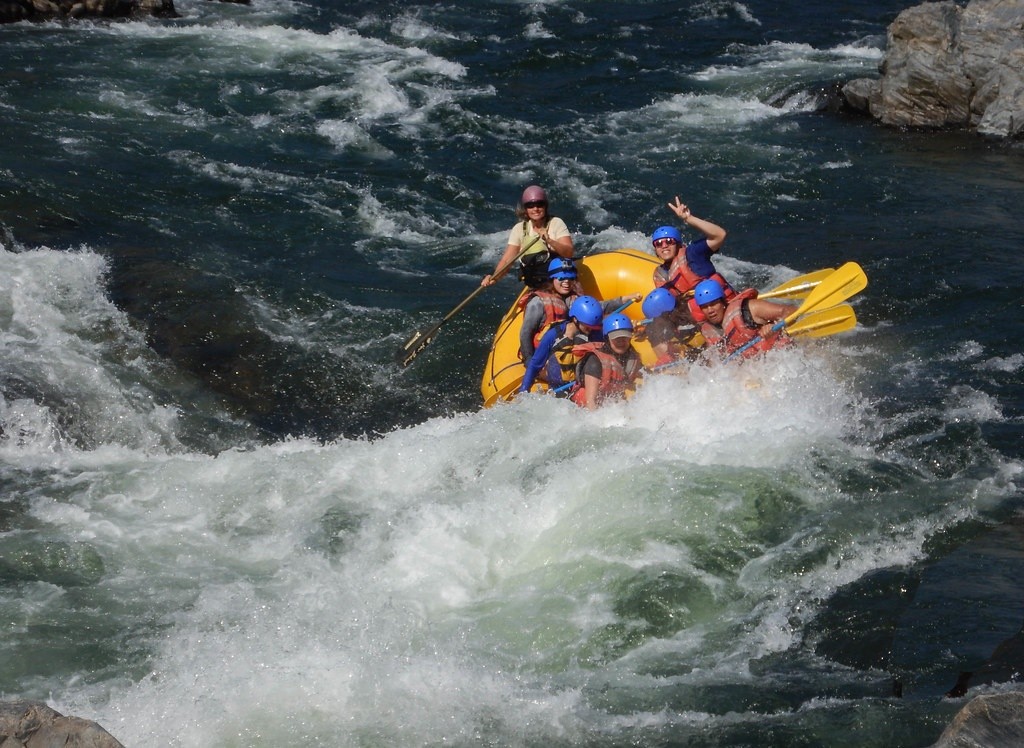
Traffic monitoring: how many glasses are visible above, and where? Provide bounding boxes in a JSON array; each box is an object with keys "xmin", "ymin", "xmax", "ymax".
[
  {"xmin": 555, "ymin": 277, "xmax": 575, "ymax": 281},
  {"xmin": 654, "ymin": 238, "xmax": 677, "ymax": 247},
  {"xmin": 525, "ymin": 202, "xmax": 546, "ymax": 208}
]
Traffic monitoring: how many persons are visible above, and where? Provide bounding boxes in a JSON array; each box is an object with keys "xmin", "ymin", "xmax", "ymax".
[
  {"xmin": 694, "ymin": 280, "xmax": 799, "ymax": 360},
  {"xmin": 481, "ymin": 185, "xmax": 574, "ymax": 292},
  {"xmin": 518, "ymin": 296, "xmax": 604, "ymax": 398},
  {"xmin": 651, "ymin": 197, "xmax": 736, "ymax": 332},
  {"xmin": 519, "ymin": 257, "xmax": 643, "ymax": 382},
  {"xmin": 642, "ymin": 288, "xmax": 713, "ymax": 372},
  {"xmin": 572, "ymin": 313, "xmax": 642, "ymax": 411}
]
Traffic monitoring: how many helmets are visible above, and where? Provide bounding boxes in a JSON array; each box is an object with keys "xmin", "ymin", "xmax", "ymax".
[
  {"xmin": 652, "ymin": 226, "xmax": 682, "ymax": 246},
  {"xmin": 569, "ymin": 295, "xmax": 603, "ymax": 326},
  {"xmin": 522, "ymin": 185, "xmax": 548, "ymax": 205},
  {"xmin": 694, "ymin": 280, "xmax": 725, "ymax": 305},
  {"xmin": 602, "ymin": 312, "xmax": 634, "ymax": 337},
  {"xmin": 642, "ymin": 287, "xmax": 676, "ymax": 319},
  {"xmin": 548, "ymin": 258, "xmax": 577, "ymax": 278}
]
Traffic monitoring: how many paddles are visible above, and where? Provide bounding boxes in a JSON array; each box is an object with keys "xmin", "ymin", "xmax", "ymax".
[
  {"xmin": 396, "ymin": 234, "xmax": 540, "ymax": 371},
  {"xmin": 722, "ymin": 261, "xmax": 868, "ymax": 365},
  {"xmin": 756, "ymin": 266, "xmax": 836, "ymax": 301},
  {"xmin": 484, "ymin": 297, "xmax": 639, "ymax": 407},
  {"xmin": 783, "ymin": 303, "xmax": 859, "ymax": 339}
]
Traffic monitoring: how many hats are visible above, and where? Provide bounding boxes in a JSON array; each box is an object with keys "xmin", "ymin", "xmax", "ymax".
[{"xmin": 608, "ymin": 329, "xmax": 633, "ymax": 339}]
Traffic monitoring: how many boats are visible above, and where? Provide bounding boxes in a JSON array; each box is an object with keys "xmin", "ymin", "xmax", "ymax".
[{"xmin": 481, "ymin": 248, "xmax": 705, "ymax": 407}]
